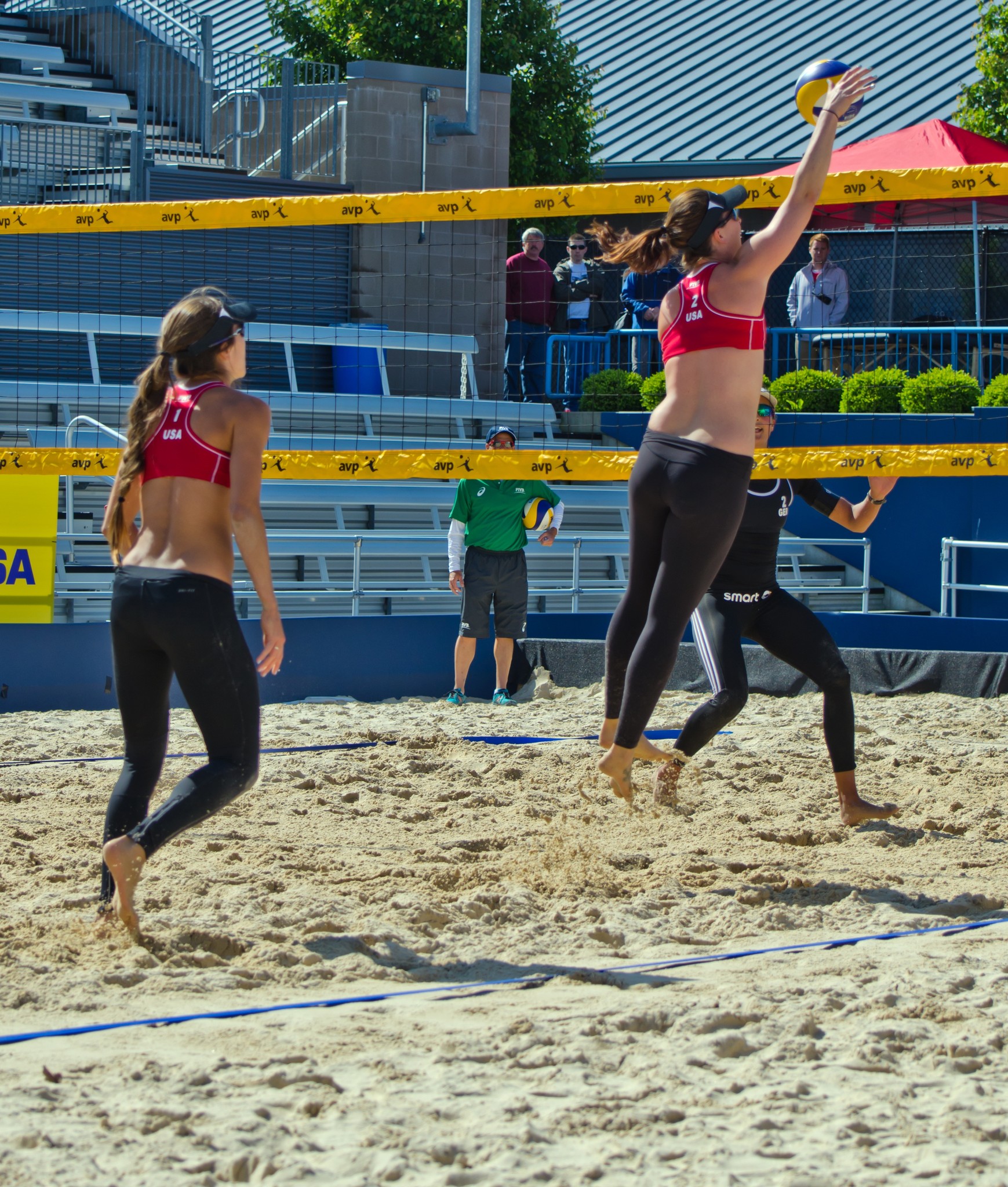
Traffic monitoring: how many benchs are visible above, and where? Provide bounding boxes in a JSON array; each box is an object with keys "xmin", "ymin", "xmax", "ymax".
[
  {"xmin": 0, "ymin": 309, "xmax": 885, "ymax": 610},
  {"xmin": 0, "ymin": 0, "xmax": 140, "ymax": 133}
]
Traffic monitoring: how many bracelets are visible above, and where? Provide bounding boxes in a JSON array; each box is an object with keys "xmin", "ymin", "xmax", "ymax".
[
  {"xmin": 867, "ymin": 492, "xmax": 887, "ymax": 505},
  {"xmin": 821, "ymin": 109, "xmax": 839, "ymax": 120}
]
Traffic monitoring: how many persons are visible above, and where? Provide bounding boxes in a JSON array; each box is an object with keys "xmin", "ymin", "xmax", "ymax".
[
  {"xmin": 94, "ymin": 286, "xmax": 286, "ymax": 942},
  {"xmin": 446, "ymin": 426, "xmax": 564, "ymax": 706},
  {"xmin": 620, "ymin": 267, "xmax": 685, "ymax": 382},
  {"xmin": 596, "ymin": 63, "xmax": 876, "ymax": 806},
  {"xmin": 654, "ymin": 387, "xmax": 899, "ymax": 823},
  {"xmin": 553, "ymin": 233, "xmax": 608, "ymax": 411},
  {"xmin": 786, "ymin": 232, "xmax": 849, "ymax": 377},
  {"xmin": 506, "ymin": 228, "xmax": 553, "ymax": 405}
]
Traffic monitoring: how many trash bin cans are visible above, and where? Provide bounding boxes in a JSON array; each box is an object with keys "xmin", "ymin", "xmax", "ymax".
[{"xmin": 328, "ymin": 323, "xmax": 389, "ymax": 396}]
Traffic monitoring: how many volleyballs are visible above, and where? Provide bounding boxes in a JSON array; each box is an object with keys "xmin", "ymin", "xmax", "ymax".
[
  {"xmin": 794, "ymin": 59, "xmax": 865, "ymax": 130},
  {"xmin": 522, "ymin": 496, "xmax": 555, "ymax": 530}
]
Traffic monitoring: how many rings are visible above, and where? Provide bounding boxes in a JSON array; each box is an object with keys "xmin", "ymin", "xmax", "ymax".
[{"xmin": 274, "ymin": 646, "xmax": 279, "ymax": 650}]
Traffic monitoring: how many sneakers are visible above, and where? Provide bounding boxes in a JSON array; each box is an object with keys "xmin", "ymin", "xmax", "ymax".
[
  {"xmin": 443, "ymin": 688, "xmax": 467, "ymax": 706},
  {"xmin": 492, "ymin": 688, "xmax": 517, "ymax": 706}
]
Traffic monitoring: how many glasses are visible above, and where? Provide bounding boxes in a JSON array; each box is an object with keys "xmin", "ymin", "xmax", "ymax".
[
  {"xmin": 211, "ymin": 322, "xmax": 246, "ymax": 348},
  {"xmin": 712, "ymin": 207, "xmax": 738, "ymax": 233},
  {"xmin": 756, "ymin": 403, "xmax": 776, "ymax": 417},
  {"xmin": 569, "ymin": 245, "xmax": 586, "ymax": 250},
  {"xmin": 487, "ymin": 439, "xmax": 515, "ymax": 450}
]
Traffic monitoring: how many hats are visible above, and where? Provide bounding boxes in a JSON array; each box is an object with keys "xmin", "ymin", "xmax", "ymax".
[
  {"xmin": 677, "ymin": 184, "xmax": 748, "ymax": 255},
  {"xmin": 760, "ymin": 387, "xmax": 778, "ymax": 410},
  {"xmin": 486, "ymin": 425, "xmax": 517, "ymax": 443},
  {"xmin": 172, "ymin": 302, "xmax": 258, "ymax": 358}
]
